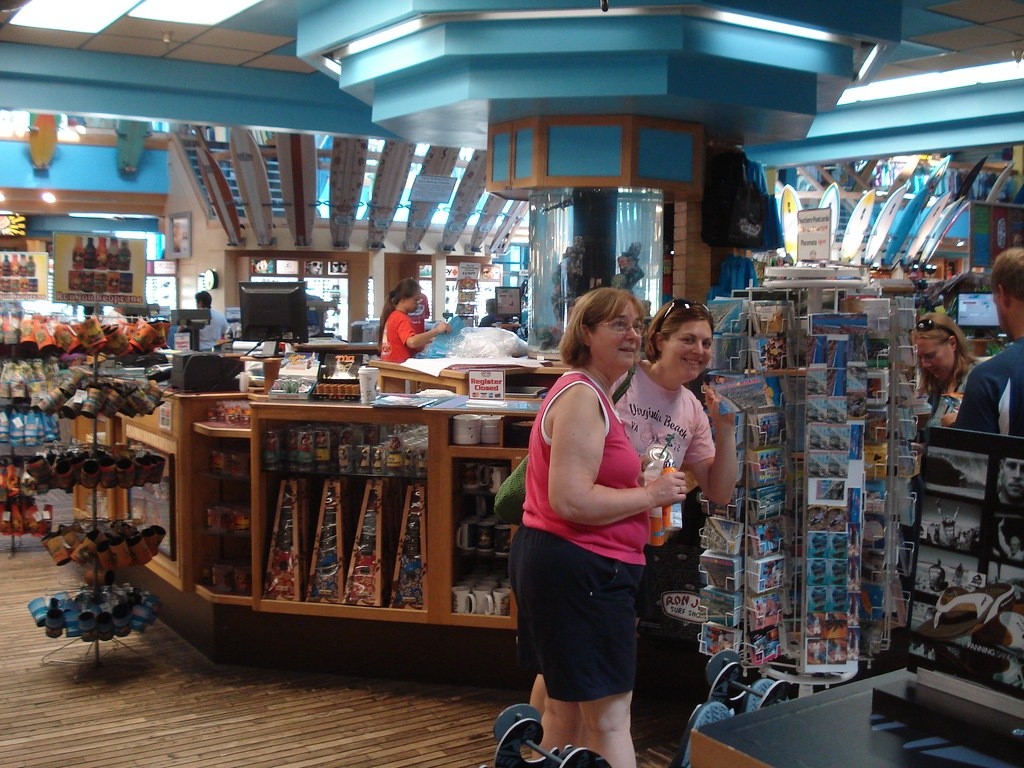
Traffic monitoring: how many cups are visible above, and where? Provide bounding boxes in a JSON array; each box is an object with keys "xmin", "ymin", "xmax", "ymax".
[
  {"xmin": 649, "ymin": 506, "xmax": 666, "ymax": 546},
  {"xmin": 357, "ymin": 366, "xmax": 379, "ymax": 405},
  {"xmin": 451, "ymin": 459, "xmax": 510, "ymax": 617}
]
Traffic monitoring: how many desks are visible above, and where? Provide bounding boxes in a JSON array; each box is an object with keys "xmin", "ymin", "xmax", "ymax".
[{"xmin": 690, "ymin": 668, "xmax": 1024, "ymax": 768}]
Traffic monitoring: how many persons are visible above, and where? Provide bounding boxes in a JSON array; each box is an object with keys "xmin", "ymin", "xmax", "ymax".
[
  {"xmin": 926, "ymin": 456, "xmax": 1024, "ymax": 591},
  {"xmin": 378, "ymin": 278, "xmax": 450, "ymax": 363},
  {"xmin": 940, "ymin": 246, "xmax": 1024, "ymax": 439},
  {"xmin": 911, "ymin": 312, "xmax": 980, "ymax": 444},
  {"xmin": 195, "ymin": 291, "xmax": 228, "ymax": 352},
  {"xmin": 528, "ymin": 297, "xmax": 737, "ymax": 713},
  {"xmin": 754, "ymin": 419, "xmax": 780, "ymax": 659},
  {"xmin": 507, "ymin": 288, "xmax": 688, "ymax": 767},
  {"xmin": 477, "ymin": 299, "xmax": 497, "ymax": 327}
]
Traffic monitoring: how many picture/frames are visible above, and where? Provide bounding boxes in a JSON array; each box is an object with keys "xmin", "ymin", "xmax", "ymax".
[{"xmin": 167, "ymin": 212, "xmax": 191, "ymax": 258}]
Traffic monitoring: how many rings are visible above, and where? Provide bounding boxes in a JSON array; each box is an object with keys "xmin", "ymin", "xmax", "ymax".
[{"xmin": 678, "ymin": 485, "xmax": 682, "ymax": 494}]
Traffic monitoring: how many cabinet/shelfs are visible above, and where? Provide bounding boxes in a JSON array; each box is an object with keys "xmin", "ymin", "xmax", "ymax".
[
  {"xmin": 33, "ymin": 306, "xmax": 170, "ymax": 683},
  {"xmin": 692, "ymin": 279, "xmax": 919, "ymax": 704},
  {"xmin": 191, "ymin": 399, "xmax": 543, "ymax": 630}
]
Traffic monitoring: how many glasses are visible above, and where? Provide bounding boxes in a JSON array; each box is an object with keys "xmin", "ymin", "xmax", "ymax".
[
  {"xmin": 916, "ymin": 319, "xmax": 957, "ymax": 337},
  {"xmin": 592, "ymin": 320, "xmax": 648, "ymax": 335},
  {"xmin": 657, "ymin": 298, "xmax": 692, "ymax": 330}
]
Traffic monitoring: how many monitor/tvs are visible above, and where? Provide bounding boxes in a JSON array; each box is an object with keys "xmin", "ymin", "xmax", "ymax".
[
  {"xmin": 956, "ymin": 291, "xmax": 1000, "ymax": 339},
  {"xmin": 238, "ymin": 281, "xmax": 309, "ymax": 345}
]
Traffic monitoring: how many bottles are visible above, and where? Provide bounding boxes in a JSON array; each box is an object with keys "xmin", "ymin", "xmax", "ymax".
[{"xmin": 660, "ymin": 458, "xmax": 682, "ymax": 532}]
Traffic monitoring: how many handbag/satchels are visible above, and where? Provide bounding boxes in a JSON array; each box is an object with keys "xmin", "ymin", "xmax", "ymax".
[{"xmin": 492, "ymin": 361, "xmax": 637, "ymax": 528}]
